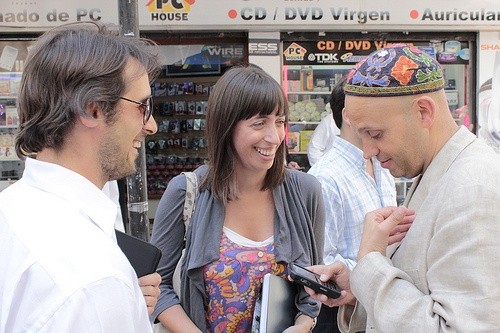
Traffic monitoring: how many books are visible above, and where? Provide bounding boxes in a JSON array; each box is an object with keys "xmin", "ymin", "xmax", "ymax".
[{"xmin": 260, "ymin": 273, "xmax": 296, "ymax": 333}]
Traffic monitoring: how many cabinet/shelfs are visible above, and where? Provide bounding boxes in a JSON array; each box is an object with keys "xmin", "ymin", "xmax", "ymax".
[{"xmin": 283, "ymin": 64, "xmax": 354, "ymax": 155}]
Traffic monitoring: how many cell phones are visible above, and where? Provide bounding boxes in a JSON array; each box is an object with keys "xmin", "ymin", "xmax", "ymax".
[{"xmin": 287, "ymin": 262, "xmax": 341, "ymax": 299}]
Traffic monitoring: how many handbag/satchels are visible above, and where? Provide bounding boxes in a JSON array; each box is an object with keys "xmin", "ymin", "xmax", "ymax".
[{"xmin": 151, "ymin": 171, "xmax": 199, "ymax": 333}]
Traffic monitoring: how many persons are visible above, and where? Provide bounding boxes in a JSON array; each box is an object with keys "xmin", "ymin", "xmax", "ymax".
[
  {"xmin": 287, "ymin": 44, "xmax": 500, "ymax": 333},
  {"xmin": 0, "ymin": 21, "xmax": 161, "ymax": 333},
  {"xmin": 148, "ymin": 63, "xmax": 326, "ymax": 333}
]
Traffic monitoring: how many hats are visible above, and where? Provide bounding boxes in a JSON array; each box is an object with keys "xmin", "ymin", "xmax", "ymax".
[{"xmin": 344, "ymin": 45, "xmax": 445, "ymax": 97}]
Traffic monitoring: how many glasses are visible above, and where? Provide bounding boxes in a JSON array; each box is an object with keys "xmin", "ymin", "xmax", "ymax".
[{"xmin": 95, "ymin": 93, "xmax": 153, "ymax": 125}]
